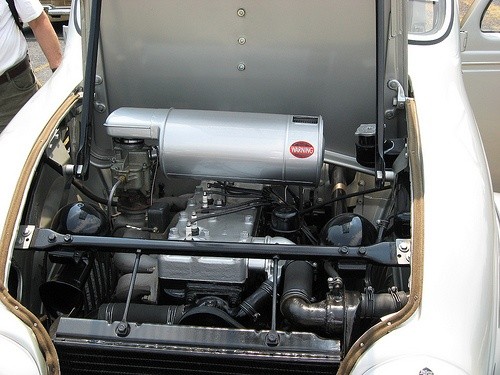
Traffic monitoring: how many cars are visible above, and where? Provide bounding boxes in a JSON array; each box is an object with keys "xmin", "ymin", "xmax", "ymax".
[{"xmin": 0, "ymin": 0, "xmax": 499, "ymax": 375}]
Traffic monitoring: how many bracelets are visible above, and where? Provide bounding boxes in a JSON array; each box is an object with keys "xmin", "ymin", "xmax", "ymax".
[{"xmin": 51, "ymin": 67, "xmax": 58, "ymax": 73}]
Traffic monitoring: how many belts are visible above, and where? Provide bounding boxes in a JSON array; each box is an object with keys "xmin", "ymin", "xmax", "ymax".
[{"xmin": 0, "ymin": 54, "xmax": 30, "ymax": 85}]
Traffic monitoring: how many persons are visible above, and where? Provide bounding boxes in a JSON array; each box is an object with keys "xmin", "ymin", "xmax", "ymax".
[{"xmin": 0, "ymin": 0, "xmax": 63, "ymax": 133}]
